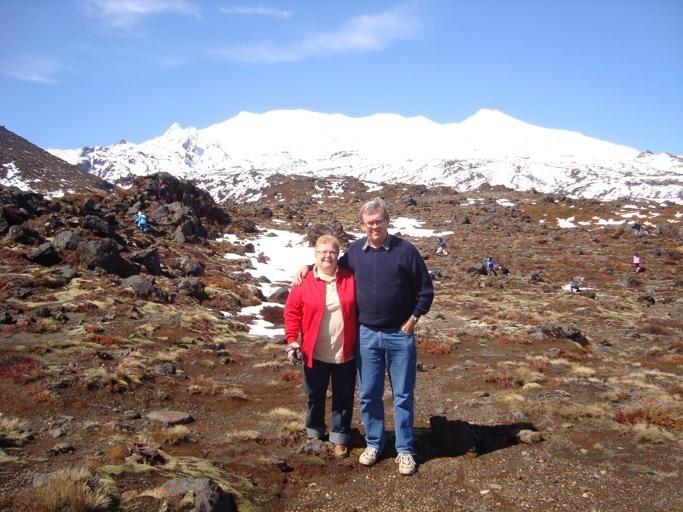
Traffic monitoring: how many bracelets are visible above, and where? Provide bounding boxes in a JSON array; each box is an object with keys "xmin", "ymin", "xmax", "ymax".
[{"xmin": 410, "ymin": 313, "xmax": 420, "ymax": 325}]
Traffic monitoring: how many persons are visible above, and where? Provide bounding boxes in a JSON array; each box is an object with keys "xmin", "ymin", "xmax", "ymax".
[
  {"xmin": 133, "ymin": 210, "xmax": 149, "ymax": 233},
  {"xmin": 632, "ymin": 252, "xmax": 641, "ymax": 272},
  {"xmin": 292, "ymin": 200, "xmax": 434, "ymax": 475},
  {"xmin": 484, "ymin": 256, "xmax": 497, "ymax": 278},
  {"xmin": 496, "ymin": 265, "xmax": 509, "ymax": 276},
  {"xmin": 282, "ymin": 234, "xmax": 357, "ymax": 459},
  {"xmin": 435, "ymin": 236, "xmax": 449, "ymax": 256}
]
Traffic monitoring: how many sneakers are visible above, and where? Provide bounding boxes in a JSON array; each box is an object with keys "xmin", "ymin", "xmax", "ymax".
[
  {"xmin": 397, "ymin": 453, "xmax": 415, "ymax": 475},
  {"xmin": 335, "ymin": 443, "xmax": 347, "ymax": 457},
  {"xmin": 359, "ymin": 445, "xmax": 379, "ymax": 466}
]
